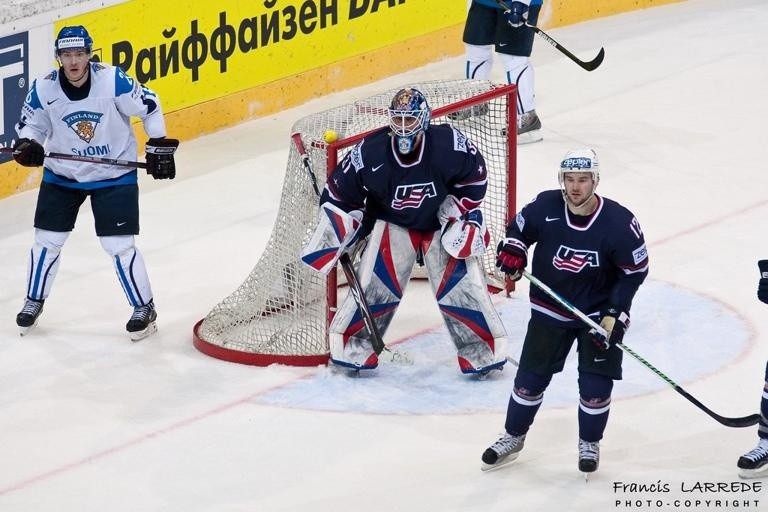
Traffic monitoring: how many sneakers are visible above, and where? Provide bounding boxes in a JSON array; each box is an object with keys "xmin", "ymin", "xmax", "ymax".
[
  {"xmin": 501, "ymin": 110, "xmax": 541, "ymax": 135},
  {"xmin": 739, "ymin": 439, "xmax": 768, "ymax": 469},
  {"xmin": 126, "ymin": 303, "xmax": 157, "ymax": 332},
  {"xmin": 482, "ymin": 434, "xmax": 526, "ymax": 464},
  {"xmin": 448, "ymin": 103, "xmax": 489, "ymax": 120},
  {"xmin": 17, "ymin": 295, "xmax": 44, "ymax": 326},
  {"xmin": 579, "ymin": 438, "xmax": 599, "ymax": 472},
  {"xmin": 589, "ymin": 310, "xmax": 631, "ymax": 351}
]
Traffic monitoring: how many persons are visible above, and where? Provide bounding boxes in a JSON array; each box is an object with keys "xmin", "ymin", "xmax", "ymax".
[
  {"xmin": 481, "ymin": 146, "xmax": 649, "ymax": 472},
  {"xmin": 738, "ymin": 255, "xmax": 768, "ymax": 469},
  {"xmin": 7, "ymin": 25, "xmax": 176, "ymax": 334},
  {"xmin": 444, "ymin": 1, "xmax": 545, "ymax": 139},
  {"xmin": 300, "ymin": 85, "xmax": 510, "ymax": 385}
]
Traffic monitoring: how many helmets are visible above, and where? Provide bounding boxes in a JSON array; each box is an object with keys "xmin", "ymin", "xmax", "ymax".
[
  {"xmin": 55, "ymin": 26, "xmax": 93, "ymax": 54},
  {"xmin": 388, "ymin": 88, "xmax": 430, "ymax": 138},
  {"xmin": 559, "ymin": 147, "xmax": 600, "ymax": 193}
]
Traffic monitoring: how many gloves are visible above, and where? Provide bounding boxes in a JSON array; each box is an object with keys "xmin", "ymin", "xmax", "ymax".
[
  {"xmin": 12, "ymin": 138, "xmax": 43, "ymax": 167},
  {"xmin": 145, "ymin": 136, "xmax": 179, "ymax": 179},
  {"xmin": 496, "ymin": 238, "xmax": 528, "ymax": 275},
  {"xmin": 504, "ymin": 1, "xmax": 530, "ymax": 27}
]
